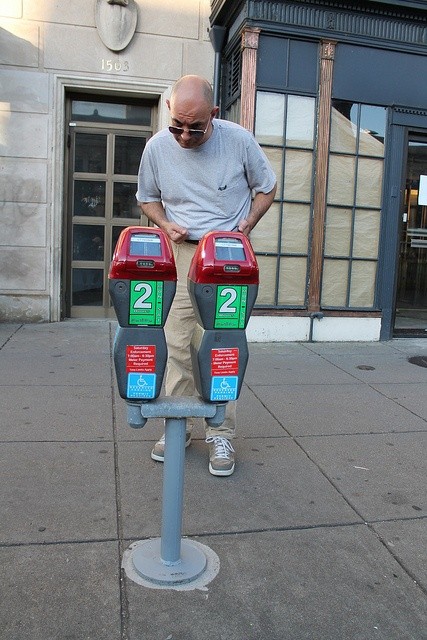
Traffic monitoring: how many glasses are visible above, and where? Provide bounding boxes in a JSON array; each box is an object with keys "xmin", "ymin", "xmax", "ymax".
[{"xmin": 169, "ymin": 114, "xmax": 212, "ymax": 139}]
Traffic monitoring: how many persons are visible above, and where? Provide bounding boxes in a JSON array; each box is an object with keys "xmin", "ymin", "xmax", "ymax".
[
  {"xmin": 134, "ymin": 74, "xmax": 278, "ymax": 477},
  {"xmin": 79, "ymin": 237, "xmax": 100, "ymax": 288}
]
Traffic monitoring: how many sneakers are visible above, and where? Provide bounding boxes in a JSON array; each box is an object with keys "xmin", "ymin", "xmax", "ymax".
[
  {"xmin": 150, "ymin": 432, "xmax": 192, "ymax": 462},
  {"xmin": 205, "ymin": 435, "xmax": 235, "ymax": 476}
]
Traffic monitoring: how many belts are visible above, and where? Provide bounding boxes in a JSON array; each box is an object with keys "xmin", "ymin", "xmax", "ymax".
[{"xmin": 185, "ymin": 240, "xmax": 199, "ymax": 245}]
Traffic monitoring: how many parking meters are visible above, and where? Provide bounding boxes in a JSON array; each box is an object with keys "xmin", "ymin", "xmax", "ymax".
[{"xmin": 108, "ymin": 226, "xmax": 259, "ymax": 586}]
